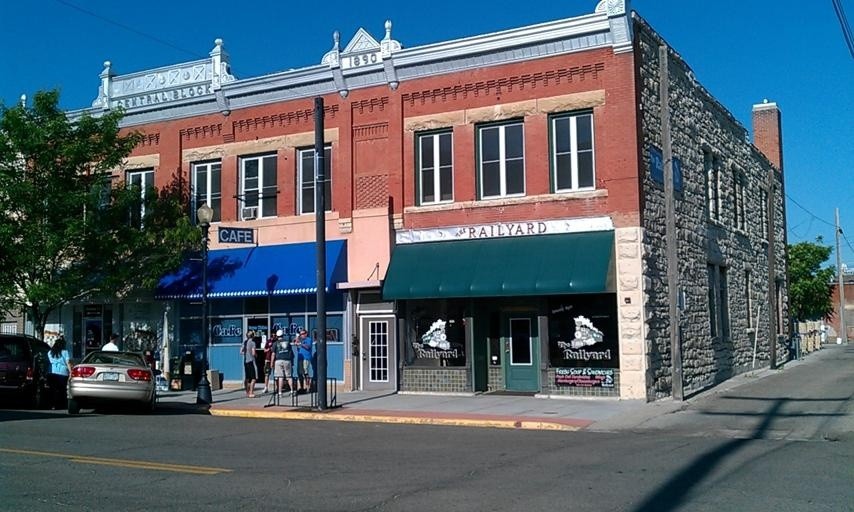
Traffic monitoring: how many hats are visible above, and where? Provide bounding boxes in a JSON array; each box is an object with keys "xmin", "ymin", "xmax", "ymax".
[{"xmin": 276, "ymin": 329, "xmax": 284, "ymax": 337}]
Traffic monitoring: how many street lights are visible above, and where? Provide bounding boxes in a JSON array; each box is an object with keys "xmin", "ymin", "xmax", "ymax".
[{"xmin": 196, "ymin": 202, "xmax": 215, "ymax": 407}]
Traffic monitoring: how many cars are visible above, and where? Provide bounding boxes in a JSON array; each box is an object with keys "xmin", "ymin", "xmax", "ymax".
[{"xmin": 65, "ymin": 349, "xmax": 159, "ymax": 415}]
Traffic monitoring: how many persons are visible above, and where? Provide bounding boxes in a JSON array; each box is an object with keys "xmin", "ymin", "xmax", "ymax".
[
  {"xmin": 101, "ymin": 334, "xmax": 120, "ymax": 352},
  {"xmin": 47, "ymin": 339, "xmax": 72, "ymax": 410},
  {"xmin": 241, "ymin": 328, "xmax": 314, "ymax": 398}
]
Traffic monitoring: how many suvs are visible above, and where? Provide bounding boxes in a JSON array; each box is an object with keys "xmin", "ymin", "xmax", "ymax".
[{"xmin": 0, "ymin": 332, "xmax": 54, "ymax": 409}]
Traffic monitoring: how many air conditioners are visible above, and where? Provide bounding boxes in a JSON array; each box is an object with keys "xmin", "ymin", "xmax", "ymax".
[{"xmin": 241, "ymin": 206, "xmax": 258, "ymax": 220}]
